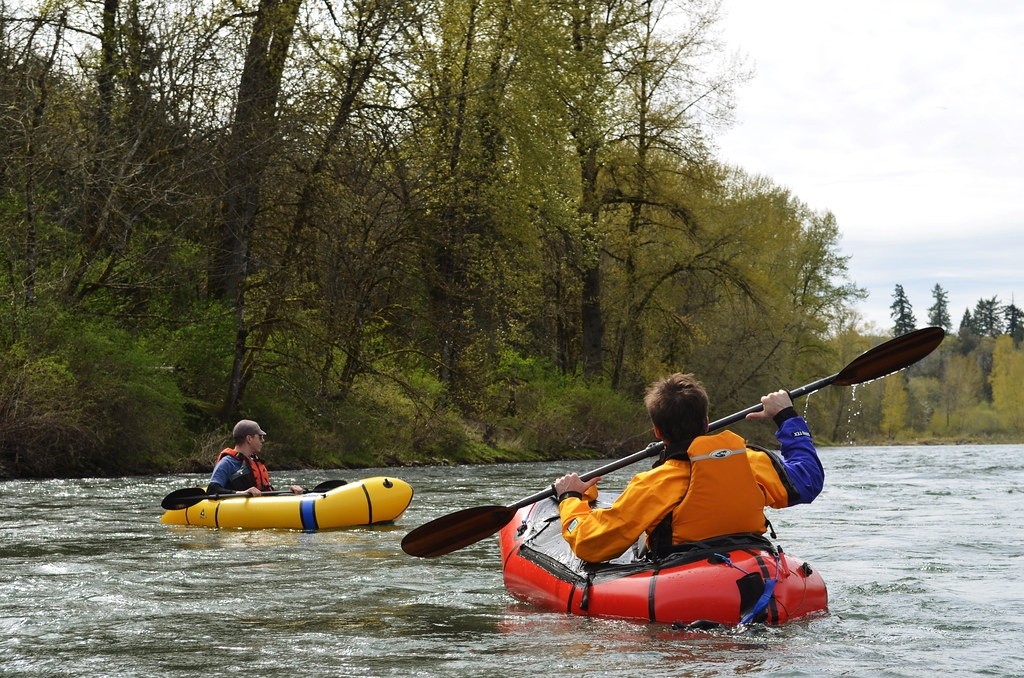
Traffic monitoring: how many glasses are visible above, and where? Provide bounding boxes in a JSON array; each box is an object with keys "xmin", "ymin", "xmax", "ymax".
[{"xmin": 250, "ymin": 435, "xmax": 262, "ymax": 439}]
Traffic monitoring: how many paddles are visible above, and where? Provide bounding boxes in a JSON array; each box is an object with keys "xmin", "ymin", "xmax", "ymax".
[
  {"xmin": 399, "ymin": 326, "xmax": 946, "ymax": 560},
  {"xmin": 161, "ymin": 479, "xmax": 348, "ymax": 514}
]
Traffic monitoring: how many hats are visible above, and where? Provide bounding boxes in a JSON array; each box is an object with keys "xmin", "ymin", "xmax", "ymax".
[{"xmin": 233, "ymin": 420, "xmax": 266, "ymax": 437}]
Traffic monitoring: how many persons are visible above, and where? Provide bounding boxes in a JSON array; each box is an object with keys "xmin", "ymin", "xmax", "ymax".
[
  {"xmin": 554, "ymin": 373, "xmax": 825, "ymax": 564},
  {"xmin": 206, "ymin": 419, "xmax": 303, "ymax": 497}
]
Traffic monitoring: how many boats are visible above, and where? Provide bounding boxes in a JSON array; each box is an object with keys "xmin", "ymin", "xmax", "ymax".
[
  {"xmin": 500, "ymin": 496, "xmax": 831, "ymax": 632},
  {"xmin": 158, "ymin": 474, "xmax": 415, "ymax": 531}
]
